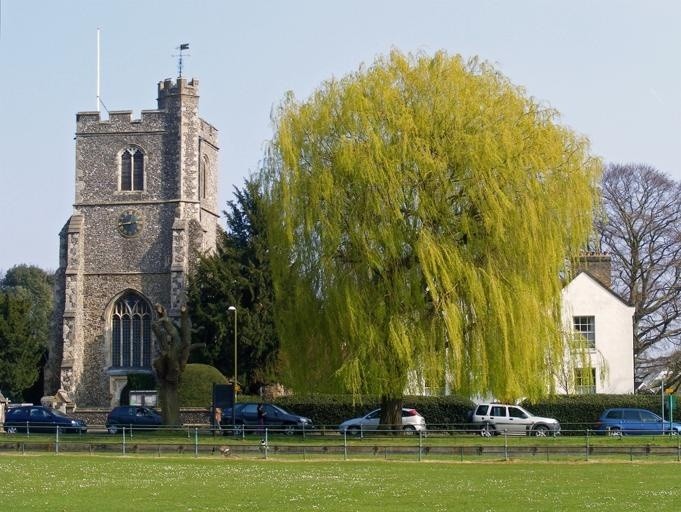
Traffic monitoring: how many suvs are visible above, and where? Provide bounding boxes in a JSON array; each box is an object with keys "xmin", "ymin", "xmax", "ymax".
[{"xmin": 467, "ymin": 403, "xmax": 561, "ymax": 436}]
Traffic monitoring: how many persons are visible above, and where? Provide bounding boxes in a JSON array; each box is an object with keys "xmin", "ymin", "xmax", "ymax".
[
  {"xmin": 214, "ymin": 408, "xmax": 222, "ymax": 436},
  {"xmin": 256, "ymin": 403, "xmax": 268, "ymax": 436}
]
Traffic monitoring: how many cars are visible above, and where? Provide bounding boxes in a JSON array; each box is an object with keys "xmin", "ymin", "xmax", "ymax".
[
  {"xmin": 105, "ymin": 406, "xmax": 161, "ymax": 434},
  {"xmin": 340, "ymin": 407, "xmax": 427, "ymax": 437},
  {"xmin": 594, "ymin": 407, "xmax": 681, "ymax": 436},
  {"xmin": 222, "ymin": 402, "xmax": 314, "ymax": 436},
  {"xmin": 3, "ymin": 406, "xmax": 87, "ymax": 433}
]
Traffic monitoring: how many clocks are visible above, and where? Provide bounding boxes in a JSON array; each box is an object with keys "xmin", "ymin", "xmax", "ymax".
[{"xmin": 117, "ymin": 208, "xmax": 146, "ymax": 238}]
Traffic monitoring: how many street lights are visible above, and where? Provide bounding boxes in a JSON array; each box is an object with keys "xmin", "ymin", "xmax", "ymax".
[{"xmin": 228, "ymin": 306, "xmax": 238, "ymax": 403}]
